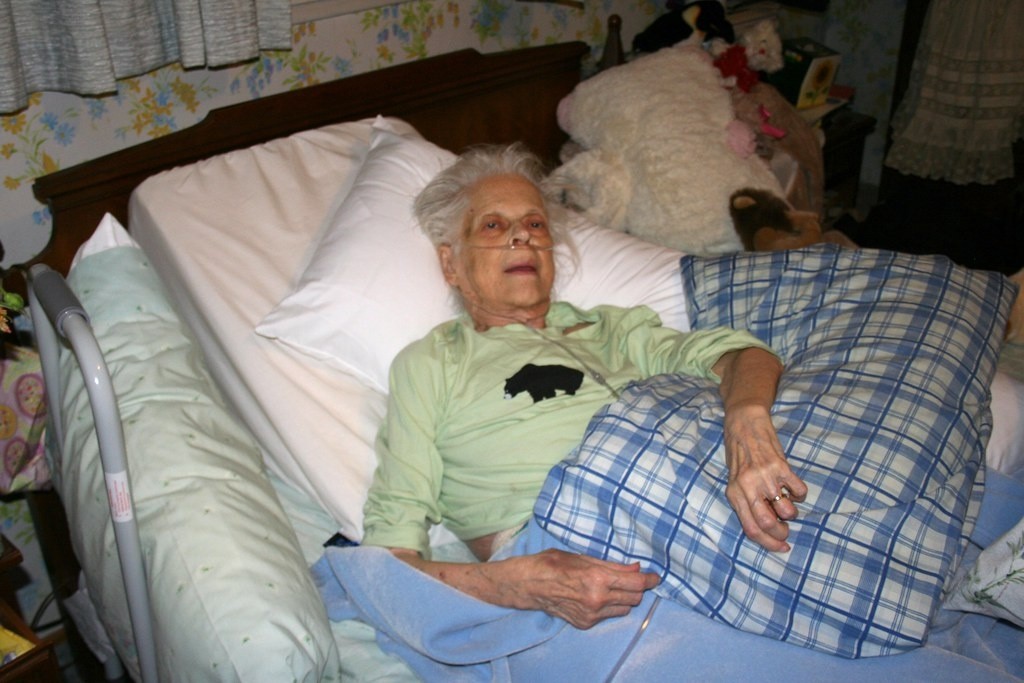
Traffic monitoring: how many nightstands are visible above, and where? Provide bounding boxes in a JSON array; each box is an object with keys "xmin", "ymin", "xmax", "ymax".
[
  {"xmin": 820, "ymin": 104, "xmax": 878, "ymax": 246},
  {"xmin": 0, "ymin": 532, "xmax": 63, "ymax": 683}
]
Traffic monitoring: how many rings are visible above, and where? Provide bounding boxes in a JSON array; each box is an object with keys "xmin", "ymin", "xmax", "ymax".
[{"xmin": 770, "ymin": 494, "xmax": 787, "ymax": 504}]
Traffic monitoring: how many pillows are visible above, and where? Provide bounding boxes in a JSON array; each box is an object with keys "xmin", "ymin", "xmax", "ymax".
[
  {"xmin": 253, "ymin": 111, "xmax": 690, "ymax": 393},
  {"xmin": 47, "ymin": 211, "xmax": 344, "ymax": 683},
  {"xmin": 127, "ymin": 118, "xmax": 459, "ymax": 547},
  {"xmin": 533, "ymin": 240, "xmax": 1020, "ymax": 660}
]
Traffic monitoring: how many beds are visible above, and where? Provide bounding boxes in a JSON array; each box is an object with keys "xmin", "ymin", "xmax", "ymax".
[{"xmin": 0, "ymin": 14, "xmax": 1024, "ymax": 683}]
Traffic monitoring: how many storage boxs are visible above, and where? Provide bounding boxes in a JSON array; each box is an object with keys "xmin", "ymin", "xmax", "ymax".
[{"xmin": 775, "ymin": 37, "xmax": 844, "ymax": 109}]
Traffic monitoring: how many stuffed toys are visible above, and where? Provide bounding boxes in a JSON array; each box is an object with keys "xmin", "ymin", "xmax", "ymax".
[{"xmin": 537, "ymin": 18, "xmax": 798, "ymax": 258}]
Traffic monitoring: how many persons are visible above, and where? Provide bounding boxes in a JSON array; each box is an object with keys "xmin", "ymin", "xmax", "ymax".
[{"xmin": 359, "ymin": 146, "xmax": 809, "ymax": 629}]
